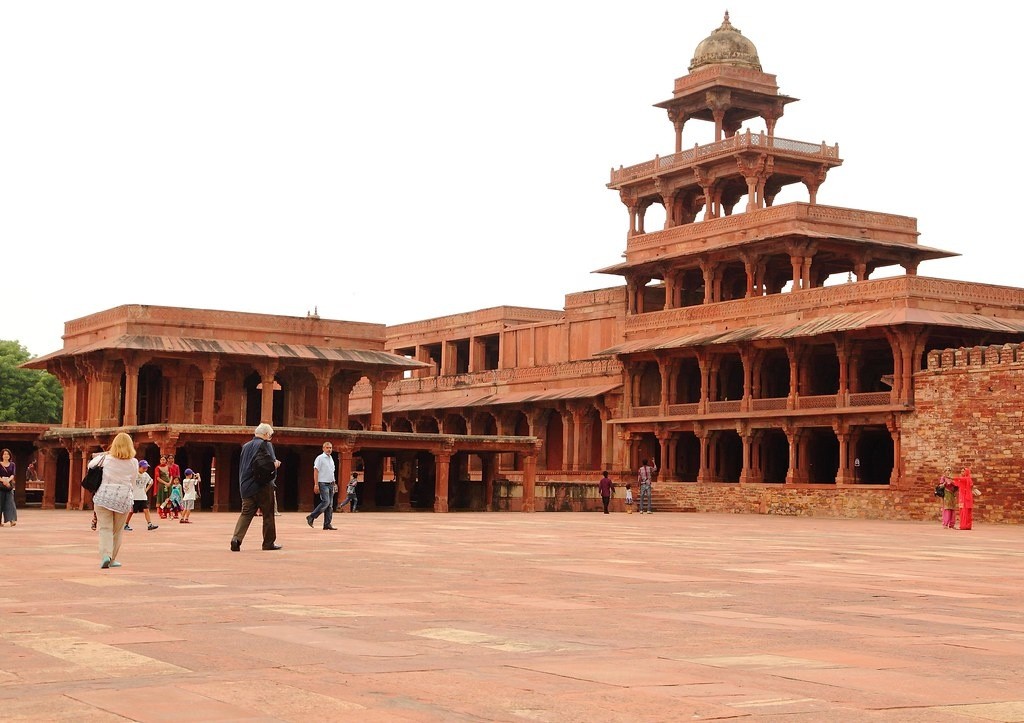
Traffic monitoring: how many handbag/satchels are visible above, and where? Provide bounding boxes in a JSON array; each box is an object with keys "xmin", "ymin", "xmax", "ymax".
[
  {"xmin": 80, "ymin": 454, "xmax": 106, "ymax": 493},
  {"xmin": 0, "ymin": 481, "xmax": 13, "ymax": 491}
]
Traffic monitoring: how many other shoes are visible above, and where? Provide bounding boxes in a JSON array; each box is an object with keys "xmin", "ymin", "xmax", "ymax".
[
  {"xmin": 109, "ymin": 560, "xmax": 121, "ymax": 567},
  {"xmin": 323, "ymin": 523, "xmax": 337, "ymax": 530},
  {"xmin": 91, "ymin": 520, "xmax": 97, "ymax": 530},
  {"xmin": 184, "ymin": 519, "xmax": 188, "ymax": 523},
  {"xmin": 306, "ymin": 515, "xmax": 314, "ymax": 528},
  {"xmin": 101, "ymin": 557, "xmax": 111, "ymax": 569},
  {"xmin": 231, "ymin": 540, "xmax": 240, "ymax": 551},
  {"xmin": 262, "ymin": 543, "xmax": 282, "ymax": 550},
  {"xmin": 647, "ymin": 511, "xmax": 652, "ymax": 514},
  {"xmin": 180, "ymin": 519, "xmax": 184, "ymax": 523},
  {"xmin": 10, "ymin": 520, "xmax": 16, "ymax": 527},
  {"xmin": 640, "ymin": 511, "xmax": 643, "ymax": 513}
]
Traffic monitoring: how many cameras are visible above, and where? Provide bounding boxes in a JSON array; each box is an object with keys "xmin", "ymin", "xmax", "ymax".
[{"xmin": 194, "ymin": 474, "xmax": 197, "ymax": 478}]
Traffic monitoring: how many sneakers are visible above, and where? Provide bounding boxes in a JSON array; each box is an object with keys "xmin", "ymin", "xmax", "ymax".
[
  {"xmin": 124, "ymin": 525, "xmax": 132, "ymax": 530},
  {"xmin": 147, "ymin": 523, "xmax": 159, "ymax": 530}
]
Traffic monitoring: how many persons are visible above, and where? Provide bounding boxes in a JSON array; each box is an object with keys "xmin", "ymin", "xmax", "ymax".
[
  {"xmin": 306, "ymin": 442, "xmax": 338, "ymax": 531},
  {"xmin": 598, "ymin": 470, "xmax": 616, "ymax": 514},
  {"xmin": 624, "ymin": 484, "xmax": 633, "ymax": 514},
  {"xmin": 153, "ymin": 454, "xmax": 201, "ymax": 522},
  {"xmin": 0, "ymin": 448, "xmax": 17, "ymax": 526},
  {"xmin": 947, "ymin": 468, "xmax": 976, "ymax": 530},
  {"xmin": 637, "ymin": 457, "xmax": 657, "ymax": 514},
  {"xmin": 91, "ymin": 460, "xmax": 158, "ymax": 531},
  {"xmin": 939, "ymin": 467, "xmax": 957, "ymax": 530},
  {"xmin": 87, "ymin": 433, "xmax": 139, "ymax": 569},
  {"xmin": 230, "ymin": 423, "xmax": 282, "ymax": 551},
  {"xmin": 337, "ymin": 472, "xmax": 359, "ymax": 512}
]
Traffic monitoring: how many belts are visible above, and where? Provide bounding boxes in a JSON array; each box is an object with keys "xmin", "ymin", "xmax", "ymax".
[{"xmin": 319, "ymin": 482, "xmax": 334, "ymax": 485}]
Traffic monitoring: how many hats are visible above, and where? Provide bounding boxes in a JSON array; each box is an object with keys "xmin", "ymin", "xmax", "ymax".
[
  {"xmin": 184, "ymin": 469, "xmax": 195, "ymax": 475},
  {"xmin": 139, "ymin": 460, "xmax": 151, "ymax": 468}
]
